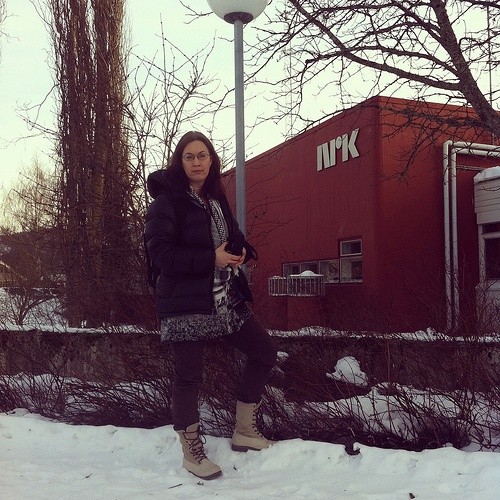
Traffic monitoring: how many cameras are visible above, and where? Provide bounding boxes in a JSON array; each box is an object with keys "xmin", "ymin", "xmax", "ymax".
[{"xmin": 225, "ymin": 239, "xmax": 243, "ymax": 258}]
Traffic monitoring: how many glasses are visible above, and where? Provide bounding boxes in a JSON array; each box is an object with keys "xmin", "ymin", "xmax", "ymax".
[{"xmin": 181, "ymin": 152, "xmax": 212, "ymax": 161}]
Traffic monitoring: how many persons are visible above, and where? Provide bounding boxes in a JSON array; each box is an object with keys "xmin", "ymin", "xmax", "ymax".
[{"xmin": 144, "ymin": 131, "xmax": 276, "ymax": 479}]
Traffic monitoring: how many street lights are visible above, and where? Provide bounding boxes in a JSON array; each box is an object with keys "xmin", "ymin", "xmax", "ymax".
[{"xmin": 204, "ymin": 1, "xmax": 269, "ymax": 277}]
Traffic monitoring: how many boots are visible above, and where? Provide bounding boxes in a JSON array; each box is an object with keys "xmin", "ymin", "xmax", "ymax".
[
  {"xmin": 231, "ymin": 399, "xmax": 269, "ymax": 452},
  {"xmin": 174, "ymin": 418, "xmax": 223, "ymax": 480}
]
